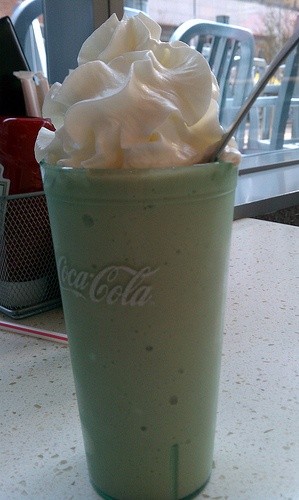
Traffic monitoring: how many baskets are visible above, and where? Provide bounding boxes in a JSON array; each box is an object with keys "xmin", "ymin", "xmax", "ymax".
[{"xmin": 0, "ymin": 164, "xmax": 62, "ymax": 319}]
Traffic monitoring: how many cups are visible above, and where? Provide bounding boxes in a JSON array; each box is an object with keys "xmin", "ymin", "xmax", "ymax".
[{"xmin": 39, "ymin": 156, "xmax": 238, "ymax": 500}]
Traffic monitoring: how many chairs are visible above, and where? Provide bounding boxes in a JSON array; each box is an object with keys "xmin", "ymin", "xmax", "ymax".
[
  {"xmin": 222, "ymin": 54, "xmax": 299, "ymax": 148},
  {"xmin": 8, "ymin": 1, "xmax": 152, "ymax": 94},
  {"xmin": 168, "ymin": 14, "xmax": 255, "ymax": 152}
]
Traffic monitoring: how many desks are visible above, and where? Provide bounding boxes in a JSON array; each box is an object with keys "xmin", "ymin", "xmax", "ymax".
[{"xmin": 1, "ymin": 214, "xmax": 298, "ymax": 498}]
